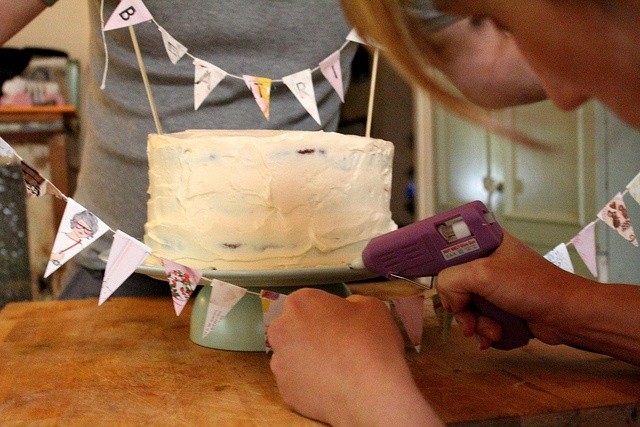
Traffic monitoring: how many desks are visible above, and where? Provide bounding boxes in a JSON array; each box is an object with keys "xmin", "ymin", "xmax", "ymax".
[
  {"xmin": 0, "ymin": 280, "xmax": 640, "ymax": 425},
  {"xmin": 2, "ymin": 105, "xmax": 77, "ymax": 298}
]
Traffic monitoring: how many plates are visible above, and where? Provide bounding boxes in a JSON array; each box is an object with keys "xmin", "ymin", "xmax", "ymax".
[{"xmin": 97, "ymin": 244, "xmax": 396, "ymax": 352}]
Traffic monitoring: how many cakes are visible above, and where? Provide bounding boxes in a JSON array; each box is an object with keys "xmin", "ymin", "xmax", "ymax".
[{"xmin": 132, "ymin": 127, "xmax": 400, "ymax": 267}]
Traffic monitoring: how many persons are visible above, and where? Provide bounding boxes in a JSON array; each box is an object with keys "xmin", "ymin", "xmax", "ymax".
[
  {"xmin": 0, "ymin": 2, "xmax": 549, "ymax": 300},
  {"xmin": 266, "ymin": 1, "xmax": 639, "ymax": 427}
]
